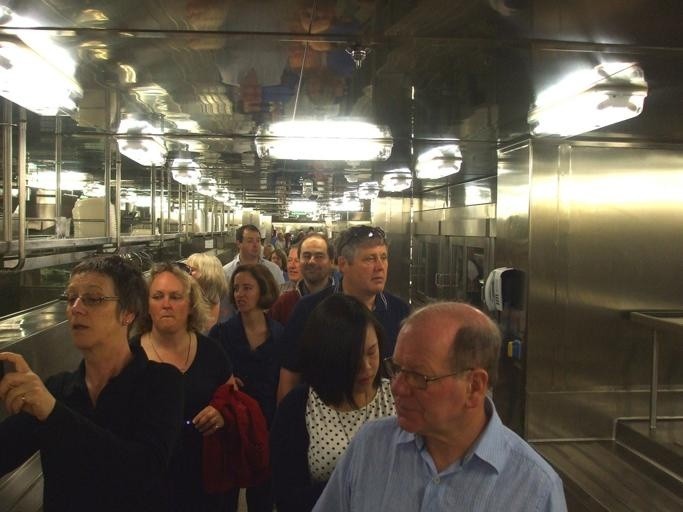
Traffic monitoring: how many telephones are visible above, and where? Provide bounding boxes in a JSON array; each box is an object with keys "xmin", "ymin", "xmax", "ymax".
[{"xmin": 483, "ymin": 267, "xmax": 524, "ymax": 313}]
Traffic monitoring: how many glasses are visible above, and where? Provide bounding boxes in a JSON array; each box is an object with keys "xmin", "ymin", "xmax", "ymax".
[
  {"xmin": 340, "ymin": 225, "xmax": 385, "ymax": 248},
  {"xmin": 64, "ymin": 289, "xmax": 120, "ymax": 308},
  {"xmin": 382, "ymin": 357, "xmax": 476, "ymax": 391}
]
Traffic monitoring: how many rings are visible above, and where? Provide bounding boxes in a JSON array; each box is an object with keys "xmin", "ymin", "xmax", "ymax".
[
  {"xmin": 213, "ymin": 424, "xmax": 223, "ymax": 432},
  {"xmin": 20, "ymin": 393, "xmax": 28, "ymax": 403}
]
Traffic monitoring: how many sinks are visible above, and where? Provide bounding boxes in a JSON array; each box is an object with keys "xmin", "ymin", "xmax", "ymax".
[{"xmin": 0, "ymin": 284, "xmax": 65, "ymax": 318}]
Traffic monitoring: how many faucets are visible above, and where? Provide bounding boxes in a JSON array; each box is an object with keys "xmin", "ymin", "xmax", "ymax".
[{"xmin": 128, "ymin": 249, "xmax": 144, "ymax": 268}]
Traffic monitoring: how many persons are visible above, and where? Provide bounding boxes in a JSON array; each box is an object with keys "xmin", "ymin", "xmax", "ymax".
[
  {"xmin": 312, "ymin": 301, "xmax": 568, "ymax": 511},
  {"xmin": 270, "ymin": 227, "xmax": 312, "ymax": 251},
  {"xmin": 219, "ymin": 263, "xmax": 285, "ymax": 510},
  {"xmin": 332, "ymin": 238, "xmax": 337, "ymax": 265},
  {"xmin": 278, "ymin": 243, "xmax": 304, "ymax": 296},
  {"xmin": 134, "ymin": 261, "xmax": 239, "ymax": 512},
  {"xmin": 0, "ymin": 253, "xmax": 184, "ymax": 512},
  {"xmin": 276, "ymin": 224, "xmax": 408, "ymax": 408},
  {"xmin": 269, "ymin": 249, "xmax": 288, "ymax": 281},
  {"xmin": 224, "ymin": 224, "xmax": 285, "ymax": 292},
  {"xmin": 327, "ymin": 238, "xmax": 334, "ymax": 265},
  {"xmin": 270, "ymin": 300, "xmax": 398, "ymax": 512},
  {"xmin": 291, "ymin": 233, "xmax": 340, "ymax": 300},
  {"xmin": 184, "ymin": 253, "xmax": 231, "ymax": 332}
]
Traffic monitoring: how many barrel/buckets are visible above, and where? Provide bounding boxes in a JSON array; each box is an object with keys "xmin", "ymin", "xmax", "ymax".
[{"xmin": 71, "ymin": 195, "xmax": 107, "ymax": 238}]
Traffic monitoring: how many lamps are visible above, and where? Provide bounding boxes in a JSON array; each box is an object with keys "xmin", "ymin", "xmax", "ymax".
[
  {"xmin": 196, "ymin": 185, "xmax": 218, "ymax": 196},
  {"xmin": 0, "ymin": 5, "xmax": 76, "ymax": 78},
  {"xmin": 196, "ymin": 178, "xmax": 219, "ymax": 190},
  {"xmin": 382, "ymin": 178, "xmax": 413, "ymax": 192},
  {"xmin": 358, "ymin": 181, "xmax": 381, "ymax": 192},
  {"xmin": 529, "ymin": 86, "xmax": 648, "ymax": 141},
  {"xmin": 113, "ymin": 134, "xmax": 168, "ymax": 167},
  {"xmin": 170, "ymin": 168, "xmax": 202, "ymax": 185},
  {"xmin": 359, "ymin": 189, "xmax": 379, "ymax": 200},
  {"xmin": 0, "ymin": 41, "xmax": 84, "ymax": 116},
  {"xmin": 170, "ymin": 158, "xmax": 202, "ymax": 178},
  {"xmin": 382, "ymin": 167, "xmax": 413, "ymax": 179},
  {"xmin": 414, "ymin": 144, "xmax": 462, "ymax": 168},
  {"xmin": 527, "ymin": 60, "xmax": 648, "ymax": 116},
  {"xmin": 255, "ymin": 120, "xmax": 393, "ymax": 141},
  {"xmin": 112, "ymin": 120, "xmax": 168, "ymax": 152},
  {"xmin": 416, "ymin": 158, "xmax": 462, "ymax": 179},
  {"xmin": 257, "ymin": 140, "xmax": 394, "ymax": 162}
]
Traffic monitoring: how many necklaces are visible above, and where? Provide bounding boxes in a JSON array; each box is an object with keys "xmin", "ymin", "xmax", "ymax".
[{"xmin": 146, "ymin": 332, "xmax": 191, "ymax": 373}]
